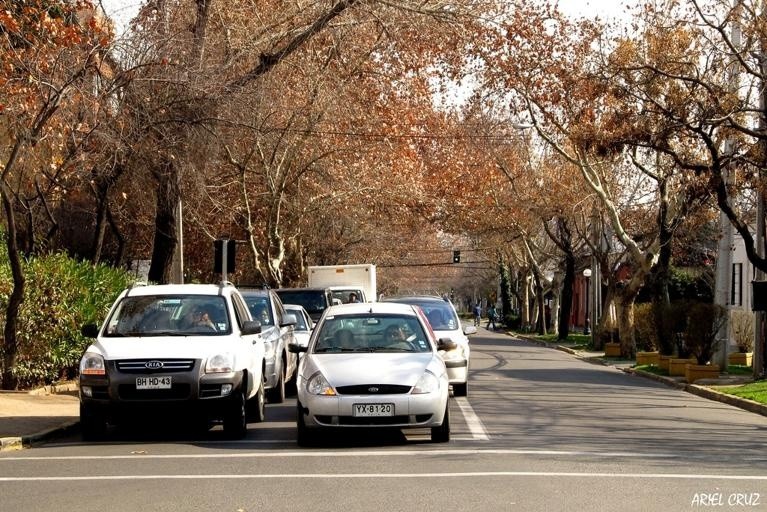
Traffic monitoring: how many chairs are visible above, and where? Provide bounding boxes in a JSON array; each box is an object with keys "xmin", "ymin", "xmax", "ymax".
[{"xmin": 332, "ymin": 328, "xmax": 353, "ymax": 349}]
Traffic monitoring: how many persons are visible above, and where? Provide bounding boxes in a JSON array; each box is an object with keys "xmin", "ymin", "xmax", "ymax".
[
  {"xmin": 428, "ymin": 307, "xmax": 446, "ymax": 329},
  {"xmin": 382, "ymin": 325, "xmax": 415, "ymax": 348},
  {"xmin": 473, "ymin": 303, "xmax": 482, "ymax": 328},
  {"xmin": 253, "ymin": 304, "xmax": 270, "ymax": 325},
  {"xmin": 192, "ymin": 302, "xmax": 221, "ymax": 330},
  {"xmin": 486, "ymin": 304, "xmax": 499, "ymax": 331},
  {"xmin": 346, "ymin": 292, "xmax": 360, "ymax": 303}
]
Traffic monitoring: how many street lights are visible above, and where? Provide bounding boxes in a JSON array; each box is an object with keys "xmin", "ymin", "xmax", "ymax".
[{"xmin": 582, "ymin": 268, "xmax": 592, "ymax": 335}]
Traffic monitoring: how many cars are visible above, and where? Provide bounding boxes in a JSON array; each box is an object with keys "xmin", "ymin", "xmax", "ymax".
[
  {"xmin": 237, "ymin": 287, "xmax": 300, "ymax": 403},
  {"xmin": 376, "ymin": 291, "xmax": 477, "ymax": 396},
  {"xmin": 282, "ymin": 303, "xmax": 317, "ymax": 360},
  {"xmin": 286, "ymin": 300, "xmax": 454, "ymax": 444}
]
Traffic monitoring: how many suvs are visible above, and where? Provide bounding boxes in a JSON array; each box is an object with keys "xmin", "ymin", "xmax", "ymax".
[{"xmin": 77, "ymin": 280, "xmax": 267, "ymax": 439}]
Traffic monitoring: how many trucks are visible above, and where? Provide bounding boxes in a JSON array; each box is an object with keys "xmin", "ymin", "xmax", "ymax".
[{"xmin": 308, "ymin": 264, "xmax": 378, "ymax": 305}]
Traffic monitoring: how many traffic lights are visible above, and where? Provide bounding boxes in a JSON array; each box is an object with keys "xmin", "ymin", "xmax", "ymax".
[{"xmin": 453, "ymin": 251, "xmax": 460, "ymax": 263}]
